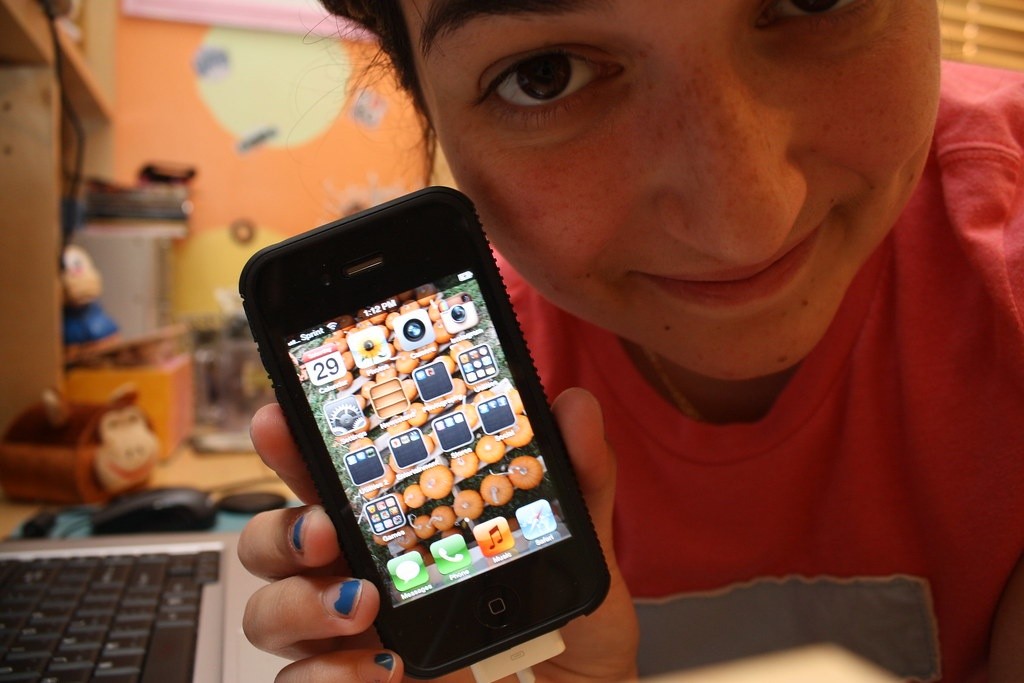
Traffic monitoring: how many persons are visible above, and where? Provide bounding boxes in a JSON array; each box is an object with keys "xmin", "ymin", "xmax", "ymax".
[{"xmin": 238, "ymin": 0, "xmax": 1021, "ymax": 683}]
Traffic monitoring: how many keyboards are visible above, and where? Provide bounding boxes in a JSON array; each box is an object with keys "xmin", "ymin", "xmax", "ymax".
[{"xmin": 1, "ymin": 533, "xmax": 272, "ymax": 683}]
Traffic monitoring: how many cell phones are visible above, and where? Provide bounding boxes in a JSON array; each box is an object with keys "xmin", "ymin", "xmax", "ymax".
[{"xmin": 238, "ymin": 185, "xmax": 612, "ymax": 680}]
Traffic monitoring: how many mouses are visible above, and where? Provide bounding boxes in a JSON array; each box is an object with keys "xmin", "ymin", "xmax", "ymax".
[{"xmin": 92, "ymin": 489, "xmax": 214, "ymax": 533}]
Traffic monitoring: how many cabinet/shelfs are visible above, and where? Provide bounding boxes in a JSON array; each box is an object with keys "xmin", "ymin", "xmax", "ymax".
[{"xmin": 0, "ymin": 0, "xmax": 114, "ymax": 485}]
